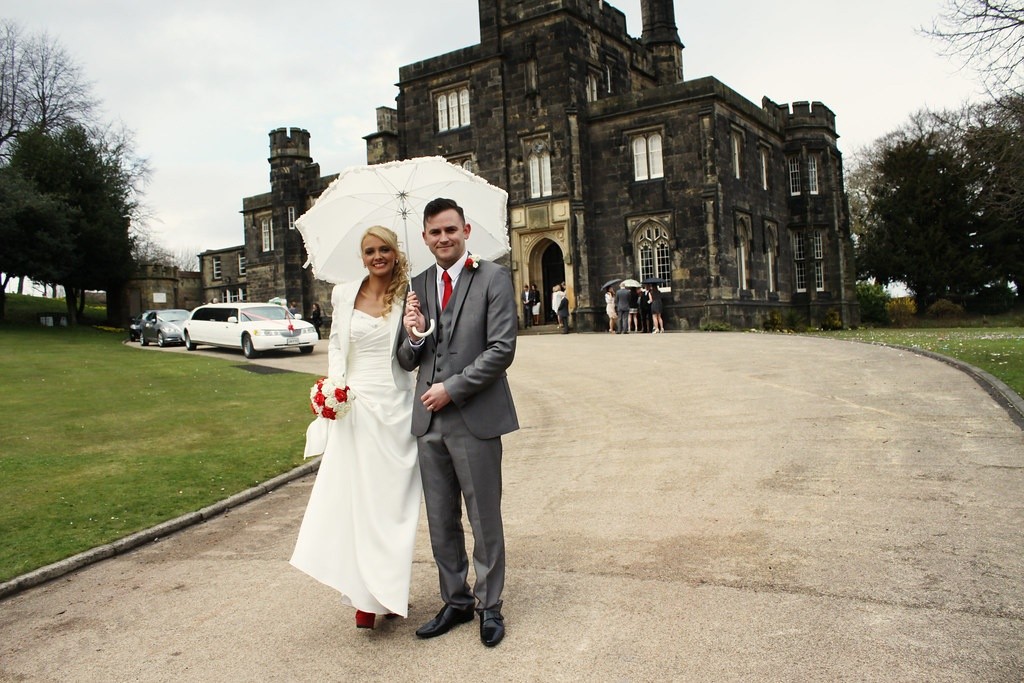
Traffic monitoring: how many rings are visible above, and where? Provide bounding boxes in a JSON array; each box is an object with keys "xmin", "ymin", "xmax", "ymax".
[{"xmin": 430, "ymin": 405, "xmax": 434, "ymax": 410}]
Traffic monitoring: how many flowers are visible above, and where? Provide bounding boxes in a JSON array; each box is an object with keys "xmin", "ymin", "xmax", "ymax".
[
  {"xmin": 465, "ymin": 255, "xmax": 480, "ymax": 269},
  {"xmin": 309, "ymin": 376, "xmax": 356, "ymax": 421}
]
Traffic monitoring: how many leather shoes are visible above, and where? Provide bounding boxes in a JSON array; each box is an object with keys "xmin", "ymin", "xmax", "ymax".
[
  {"xmin": 478, "ymin": 607, "xmax": 505, "ymax": 647},
  {"xmin": 414, "ymin": 602, "xmax": 475, "ymax": 638}
]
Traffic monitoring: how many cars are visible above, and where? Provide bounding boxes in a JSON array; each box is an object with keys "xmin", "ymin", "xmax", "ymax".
[
  {"xmin": 182, "ymin": 301, "xmax": 318, "ymax": 359},
  {"xmin": 128, "ymin": 309, "xmax": 156, "ymax": 342},
  {"xmin": 139, "ymin": 309, "xmax": 191, "ymax": 347}
]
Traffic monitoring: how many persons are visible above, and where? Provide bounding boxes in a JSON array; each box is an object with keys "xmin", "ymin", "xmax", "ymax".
[
  {"xmin": 312, "ymin": 303, "xmax": 322, "ymax": 339},
  {"xmin": 288, "ymin": 302, "xmax": 298, "ymax": 315},
  {"xmin": 551, "ymin": 281, "xmax": 570, "ymax": 334},
  {"xmin": 522, "ymin": 284, "xmax": 541, "ymax": 330},
  {"xmin": 605, "ymin": 283, "xmax": 664, "ymax": 333},
  {"xmin": 396, "ymin": 198, "xmax": 522, "ymax": 648},
  {"xmin": 287, "ymin": 225, "xmax": 422, "ymax": 631}
]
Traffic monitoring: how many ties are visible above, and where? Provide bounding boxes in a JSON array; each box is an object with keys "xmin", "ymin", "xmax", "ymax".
[{"xmin": 441, "ymin": 270, "xmax": 454, "ymax": 312}]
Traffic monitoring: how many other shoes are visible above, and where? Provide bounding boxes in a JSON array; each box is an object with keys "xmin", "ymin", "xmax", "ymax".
[
  {"xmin": 623, "ymin": 332, "xmax": 629, "ymax": 334},
  {"xmin": 652, "ymin": 329, "xmax": 660, "ymax": 334},
  {"xmin": 355, "ymin": 610, "xmax": 377, "ymax": 631},
  {"xmin": 615, "ymin": 331, "xmax": 620, "ymax": 334},
  {"xmin": 660, "ymin": 328, "xmax": 665, "ymax": 333},
  {"xmin": 609, "ymin": 329, "xmax": 615, "ymax": 334},
  {"xmin": 385, "ymin": 612, "xmax": 399, "ymax": 619}
]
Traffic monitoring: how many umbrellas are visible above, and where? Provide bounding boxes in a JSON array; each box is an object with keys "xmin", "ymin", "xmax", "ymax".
[
  {"xmin": 640, "ymin": 277, "xmax": 665, "ymax": 284},
  {"xmin": 291, "ymin": 154, "xmax": 511, "ymax": 339},
  {"xmin": 619, "ymin": 279, "xmax": 642, "ymax": 288},
  {"xmin": 600, "ymin": 278, "xmax": 622, "ymax": 293}
]
